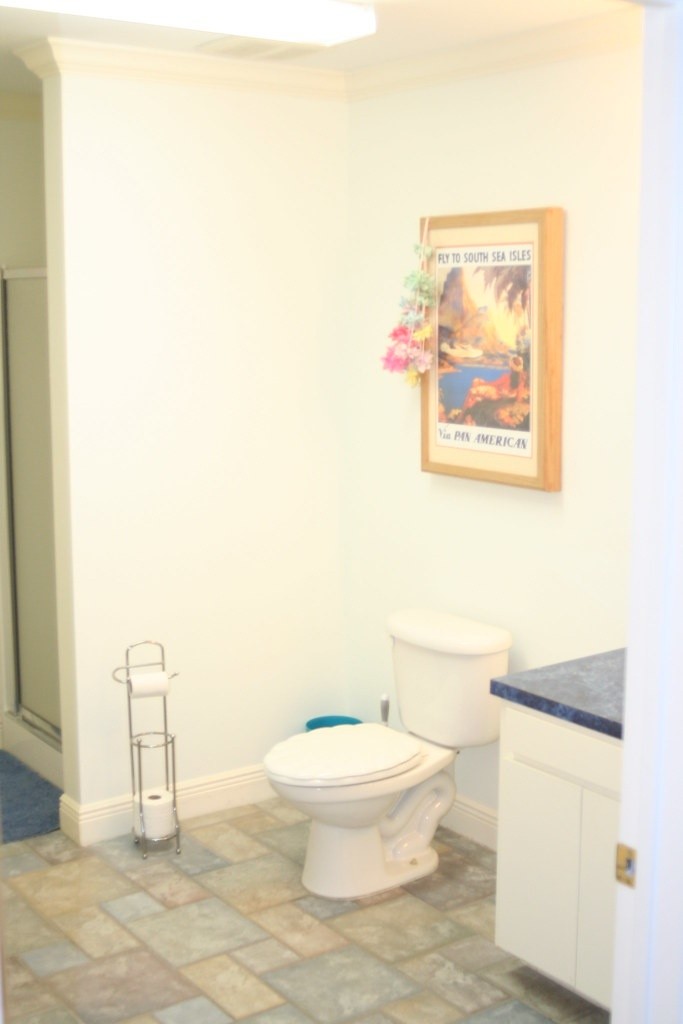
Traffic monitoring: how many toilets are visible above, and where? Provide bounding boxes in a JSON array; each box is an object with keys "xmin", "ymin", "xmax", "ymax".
[{"xmin": 262, "ymin": 605, "xmax": 512, "ymax": 902}]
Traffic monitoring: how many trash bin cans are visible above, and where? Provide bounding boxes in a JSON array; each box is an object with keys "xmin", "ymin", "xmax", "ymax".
[{"xmin": 306, "ymin": 714, "xmax": 363, "ymax": 733}]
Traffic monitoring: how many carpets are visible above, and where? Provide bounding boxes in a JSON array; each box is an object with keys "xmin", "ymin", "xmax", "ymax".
[{"xmin": 0, "ymin": 747, "xmax": 64, "ymax": 847}]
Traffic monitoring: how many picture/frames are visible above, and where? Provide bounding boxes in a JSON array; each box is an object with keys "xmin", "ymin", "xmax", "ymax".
[{"xmin": 415, "ymin": 206, "xmax": 567, "ymax": 494}]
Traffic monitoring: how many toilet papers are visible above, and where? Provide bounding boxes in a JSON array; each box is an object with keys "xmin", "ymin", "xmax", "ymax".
[
  {"xmin": 133, "ymin": 788, "xmax": 174, "ymax": 840},
  {"xmin": 128, "ymin": 671, "xmax": 169, "ymax": 698}
]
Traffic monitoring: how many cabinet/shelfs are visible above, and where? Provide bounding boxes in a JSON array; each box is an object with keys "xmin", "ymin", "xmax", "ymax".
[{"xmin": 492, "ymin": 700, "xmax": 624, "ymax": 1013}]
